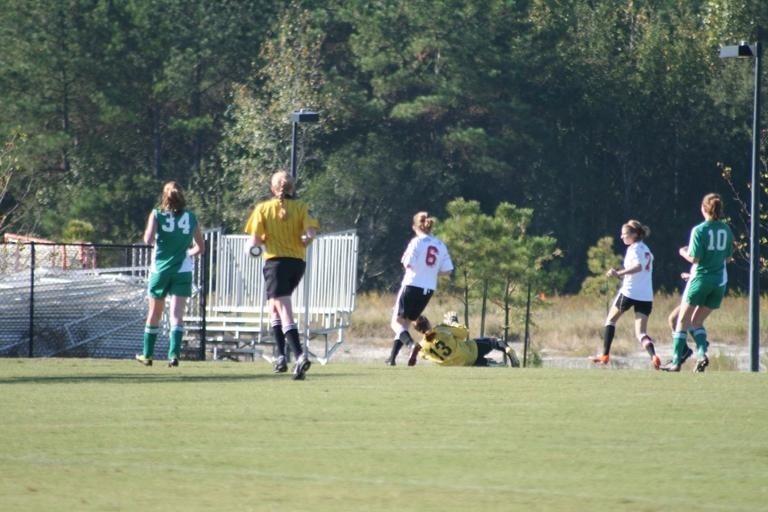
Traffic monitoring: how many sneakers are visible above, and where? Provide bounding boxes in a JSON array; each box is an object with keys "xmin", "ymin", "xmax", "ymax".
[
  {"xmin": 291, "ymin": 353, "xmax": 311, "ymax": 380},
  {"xmin": 652, "ymin": 346, "xmax": 709, "ymax": 372},
  {"xmin": 135, "ymin": 353, "xmax": 152, "ymax": 366},
  {"xmin": 505, "ymin": 347, "xmax": 520, "ymax": 367},
  {"xmin": 408, "ymin": 343, "xmax": 421, "ymax": 366},
  {"xmin": 272, "ymin": 355, "xmax": 287, "ymax": 372},
  {"xmin": 168, "ymin": 359, "xmax": 178, "ymax": 367},
  {"xmin": 588, "ymin": 355, "xmax": 609, "ymax": 365}
]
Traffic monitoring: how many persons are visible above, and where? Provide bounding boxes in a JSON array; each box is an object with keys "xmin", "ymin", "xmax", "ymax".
[
  {"xmin": 412, "ymin": 313, "xmax": 521, "ymax": 368},
  {"xmin": 384, "ymin": 210, "xmax": 456, "ymax": 366},
  {"xmin": 662, "ymin": 273, "xmax": 710, "ymax": 370},
  {"xmin": 245, "ymin": 172, "xmax": 319, "ymax": 381},
  {"xmin": 134, "ymin": 180, "xmax": 205, "ymax": 368},
  {"xmin": 589, "ymin": 219, "xmax": 660, "ymax": 368},
  {"xmin": 665, "ymin": 194, "xmax": 735, "ymax": 372}
]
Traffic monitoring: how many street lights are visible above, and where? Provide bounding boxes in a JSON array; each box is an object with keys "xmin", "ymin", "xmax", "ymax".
[
  {"xmin": 716, "ymin": 38, "xmax": 766, "ymax": 373},
  {"xmin": 287, "ymin": 106, "xmax": 321, "ymax": 178}
]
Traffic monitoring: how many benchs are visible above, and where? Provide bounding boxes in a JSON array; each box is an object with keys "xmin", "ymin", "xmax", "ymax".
[{"xmin": 181, "ymin": 304, "xmax": 300, "ymax": 363}]
